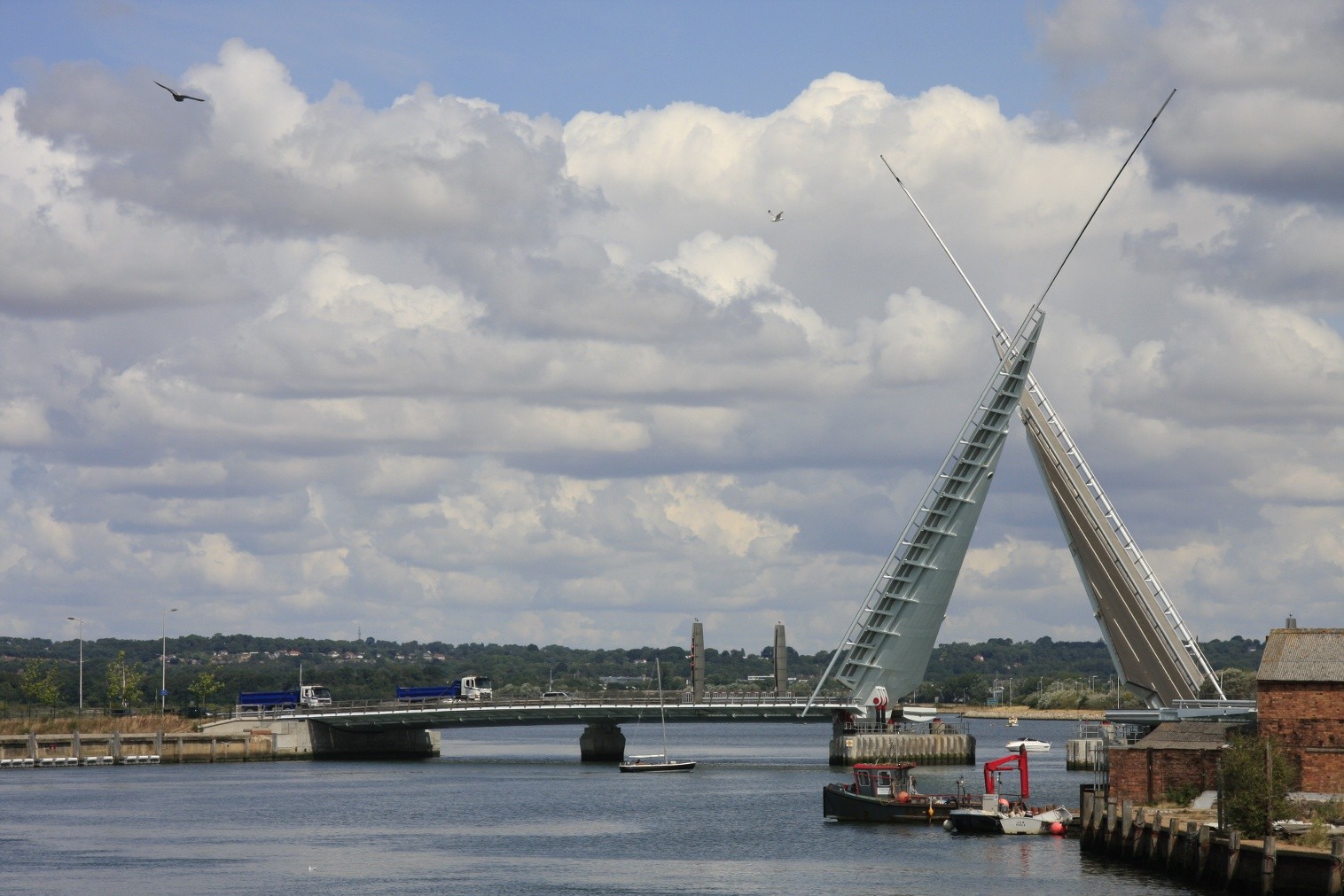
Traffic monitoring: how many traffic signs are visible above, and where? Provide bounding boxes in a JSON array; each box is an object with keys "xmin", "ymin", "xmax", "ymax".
[{"xmin": 160, "ymin": 690, "xmax": 168, "ymax": 696}]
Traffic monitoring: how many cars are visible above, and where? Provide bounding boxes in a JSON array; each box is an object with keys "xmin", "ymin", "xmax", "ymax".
[{"xmin": 177, "ymin": 707, "xmax": 221, "ymax": 718}]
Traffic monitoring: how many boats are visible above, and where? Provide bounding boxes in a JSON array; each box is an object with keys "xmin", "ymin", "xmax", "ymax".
[
  {"xmin": 1005, "ymin": 738, "xmax": 1051, "ymax": 752},
  {"xmin": 949, "ymin": 794, "xmax": 1073, "ymax": 836},
  {"xmin": 822, "ymin": 723, "xmax": 1026, "ymax": 821},
  {"xmin": 1005, "ymin": 712, "xmax": 1019, "ymax": 727}
]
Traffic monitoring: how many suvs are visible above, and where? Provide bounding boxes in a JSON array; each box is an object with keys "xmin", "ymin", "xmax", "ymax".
[{"xmin": 539, "ymin": 691, "xmax": 575, "ymax": 699}]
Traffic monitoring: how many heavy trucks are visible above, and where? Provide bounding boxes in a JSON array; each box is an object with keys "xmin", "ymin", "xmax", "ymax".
[
  {"xmin": 239, "ymin": 685, "xmax": 332, "ymax": 715},
  {"xmin": 397, "ymin": 676, "xmax": 493, "ymax": 707}
]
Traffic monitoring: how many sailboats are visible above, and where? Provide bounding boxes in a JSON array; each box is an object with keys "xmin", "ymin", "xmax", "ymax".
[{"xmin": 618, "ymin": 657, "xmax": 696, "ymax": 774}]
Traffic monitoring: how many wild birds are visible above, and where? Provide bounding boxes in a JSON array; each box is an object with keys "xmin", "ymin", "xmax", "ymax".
[
  {"xmin": 151, "ymin": 79, "xmax": 205, "ymax": 102},
  {"xmin": 768, "ymin": 209, "xmax": 784, "ymax": 222}
]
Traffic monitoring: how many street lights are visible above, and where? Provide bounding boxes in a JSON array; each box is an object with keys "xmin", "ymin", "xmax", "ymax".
[
  {"xmin": 993, "ymin": 680, "xmax": 996, "ymax": 699},
  {"xmin": 1052, "ymin": 672, "xmax": 1120, "ymax": 709},
  {"xmin": 161, "ymin": 608, "xmax": 179, "ymax": 716},
  {"xmin": 66, "ymin": 617, "xmax": 84, "ymax": 718},
  {"xmin": 995, "ymin": 678, "xmax": 999, "ymax": 699},
  {"xmin": 963, "ymin": 688, "xmax": 968, "ymax": 704},
  {"xmin": 1009, "ymin": 678, "xmax": 1013, "ymax": 706},
  {"xmin": 1037, "ymin": 681, "xmax": 1042, "ymax": 696},
  {"xmin": 1041, "ymin": 677, "xmax": 1044, "ymax": 696}
]
